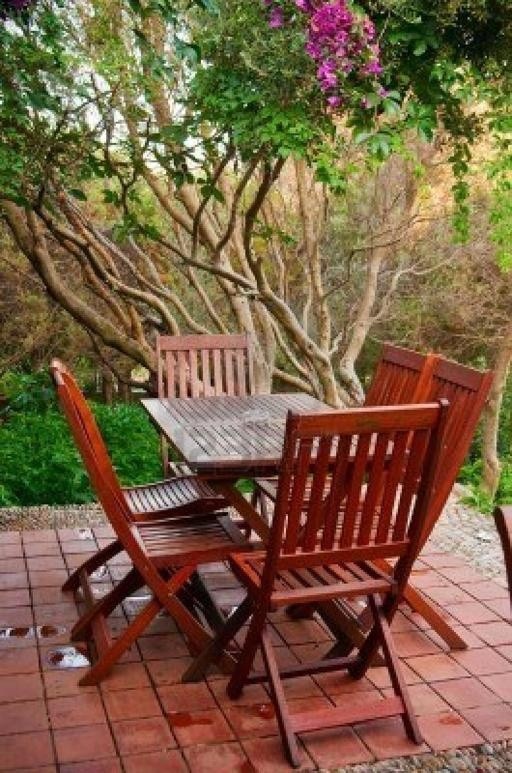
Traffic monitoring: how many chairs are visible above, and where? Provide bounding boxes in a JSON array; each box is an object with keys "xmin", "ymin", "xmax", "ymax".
[
  {"xmin": 254, "ymin": 343, "xmax": 438, "ymax": 515},
  {"xmin": 49, "ymin": 356, "xmax": 228, "ymax": 634},
  {"xmin": 299, "ymin": 353, "xmax": 496, "ymax": 660},
  {"xmin": 228, "ymin": 396, "xmax": 451, "ymax": 768},
  {"xmin": 52, "ymin": 370, "xmax": 254, "ymax": 686},
  {"xmin": 492, "ymin": 505, "xmax": 511, "ymax": 608},
  {"xmin": 155, "ymin": 332, "xmax": 269, "ymax": 542}
]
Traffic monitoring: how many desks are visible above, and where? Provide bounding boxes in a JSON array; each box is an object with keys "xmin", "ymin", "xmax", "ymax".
[{"xmin": 140, "ymin": 393, "xmax": 411, "ymax": 682}]
